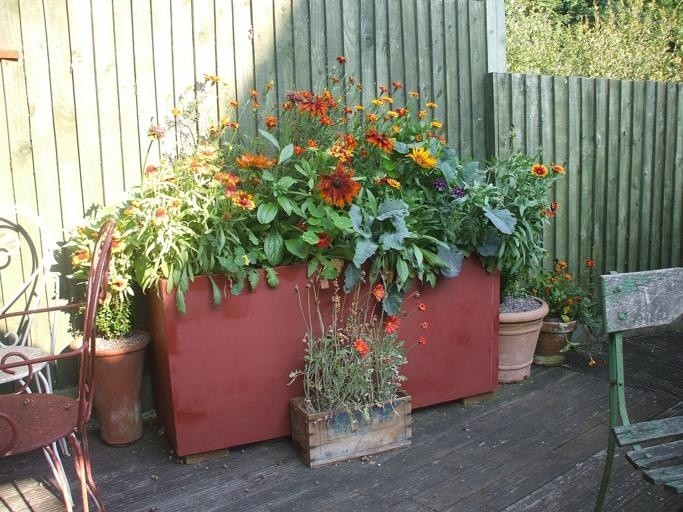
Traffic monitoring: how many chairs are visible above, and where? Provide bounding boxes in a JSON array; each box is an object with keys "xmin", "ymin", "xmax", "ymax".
[
  {"xmin": 0, "ymin": 202, "xmax": 72, "ymax": 460},
  {"xmin": 1, "ymin": 217, "xmax": 114, "ymax": 512},
  {"xmin": 593, "ymin": 265, "xmax": 682, "ymax": 511}
]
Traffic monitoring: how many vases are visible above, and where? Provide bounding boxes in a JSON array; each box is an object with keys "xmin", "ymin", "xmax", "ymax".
[
  {"xmin": 533, "ymin": 315, "xmax": 583, "ymax": 369},
  {"xmin": 490, "ymin": 291, "xmax": 549, "ymax": 385},
  {"xmin": 286, "ymin": 388, "xmax": 418, "ymax": 470},
  {"xmin": 75, "ymin": 329, "xmax": 155, "ymax": 448}
]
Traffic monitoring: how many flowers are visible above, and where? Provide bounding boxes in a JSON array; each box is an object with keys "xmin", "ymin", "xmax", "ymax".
[
  {"xmin": 525, "ymin": 157, "xmax": 573, "ymax": 226},
  {"xmin": 531, "ymin": 248, "xmax": 593, "ymax": 328},
  {"xmin": 69, "ymin": 207, "xmax": 140, "ymax": 314},
  {"xmin": 122, "ymin": 53, "xmax": 470, "ymax": 248},
  {"xmin": 285, "ymin": 276, "xmax": 432, "ymax": 406}
]
